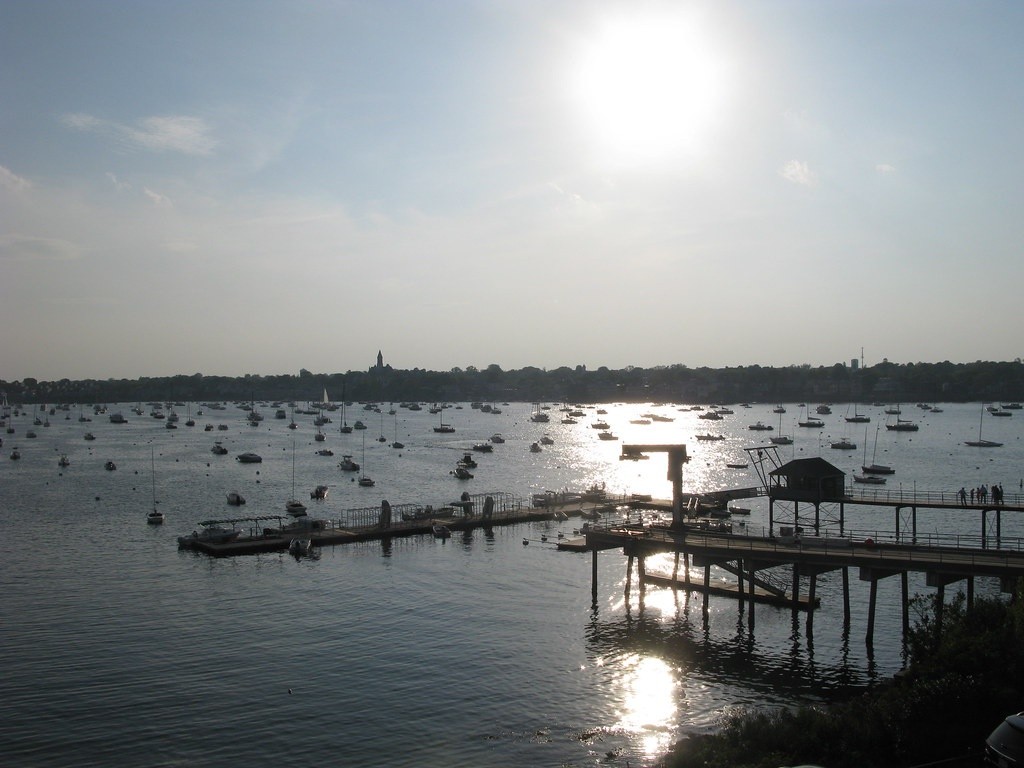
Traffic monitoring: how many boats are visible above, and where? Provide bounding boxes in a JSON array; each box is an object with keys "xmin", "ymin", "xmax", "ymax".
[
  {"xmin": 730, "ymin": 506, "xmax": 752, "ymax": 515},
  {"xmin": 401, "ymin": 506, "xmax": 453, "ymax": 520},
  {"xmin": 533, "ymin": 490, "xmax": 584, "ymax": 507},
  {"xmin": 434, "ymin": 522, "xmax": 453, "ymax": 539},
  {"xmin": 177, "ymin": 523, "xmax": 240, "ymax": 547},
  {"xmin": 581, "ymin": 508, "xmax": 601, "ymax": 518},
  {"xmin": 264, "ymin": 517, "xmax": 326, "ymax": 538},
  {"xmin": 727, "ymin": 464, "xmax": 748, "ymax": 469},
  {"xmin": 288, "ymin": 538, "xmax": 311, "ymax": 555},
  {"xmin": 0, "ymin": 396, "xmax": 734, "ymax": 525},
  {"xmin": 553, "ymin": 510, "xmax": 569, "ymax": 523}
]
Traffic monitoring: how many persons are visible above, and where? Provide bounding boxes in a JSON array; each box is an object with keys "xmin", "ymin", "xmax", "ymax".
[
  {"xmin": 976, "ymin": 484, "xmax": 1004, "ymax": 505},
  {"xmin": 793, "ymin": 531, "xmax": 802, "ymax": 546},
  {"xmin": 958, "ymin": 488, "xmax": 969, "ymax": 505},
  {"xmin": 970, "ymin": 489, "xmax": 975, "ymax": 503},
  {"xmin": 591, "ymin": 482, "xmax": 606, "ymax": 491}
]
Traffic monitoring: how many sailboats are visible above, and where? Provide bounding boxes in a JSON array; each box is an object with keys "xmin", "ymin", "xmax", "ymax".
[{"xmin": 749, "ymin": 379, "xmax": 1023, "ymax": 485}]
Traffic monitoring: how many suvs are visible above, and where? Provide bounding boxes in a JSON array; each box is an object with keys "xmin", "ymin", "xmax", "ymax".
[{"xmin": 983, "ymin": 712, "xmax": 1024, "ymax": 768}]
